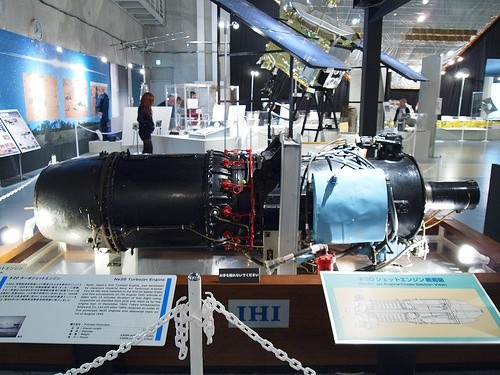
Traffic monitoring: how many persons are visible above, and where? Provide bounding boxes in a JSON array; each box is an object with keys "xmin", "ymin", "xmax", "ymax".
[
  {"xmin": 96, "ymin": 88, "xmax": 109, "ymax": 133},
  {"xmin": 137, "ymin": 92, "xmax": 154, "ymax": 154},
  {"xmin": 157, "ymin": 95, "xmax": 175, "ymax": 106},
  {"xmin": 270, "ymin": 104, "xmax": 280, "ymax": 118},
  {"xmin": 393, "ymin": 99, "xmax": 410, "ymax": 131},
  {"xmin": 191, "ymin": 91, "xmax": 196, "ymax": 98},
  {"xmin": 177, "ymin": 96, "xmax": 184, "ymax": 107}
]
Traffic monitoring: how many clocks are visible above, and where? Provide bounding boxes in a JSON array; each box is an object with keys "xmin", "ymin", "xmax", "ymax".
[{"xmin": 30, "ymin": 19, "xmax": 43, "ymax": 42}]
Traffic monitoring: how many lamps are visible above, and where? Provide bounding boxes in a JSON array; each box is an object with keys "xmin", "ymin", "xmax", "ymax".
[{"xmin": 229, "ymin": 21, "xmax": 239, "ymax": 30}]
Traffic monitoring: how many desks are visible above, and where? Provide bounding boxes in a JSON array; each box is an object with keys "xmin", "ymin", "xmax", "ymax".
[{"xmin": 150, "ymin": 133, "xmax": 241, "ymax": 153}]
